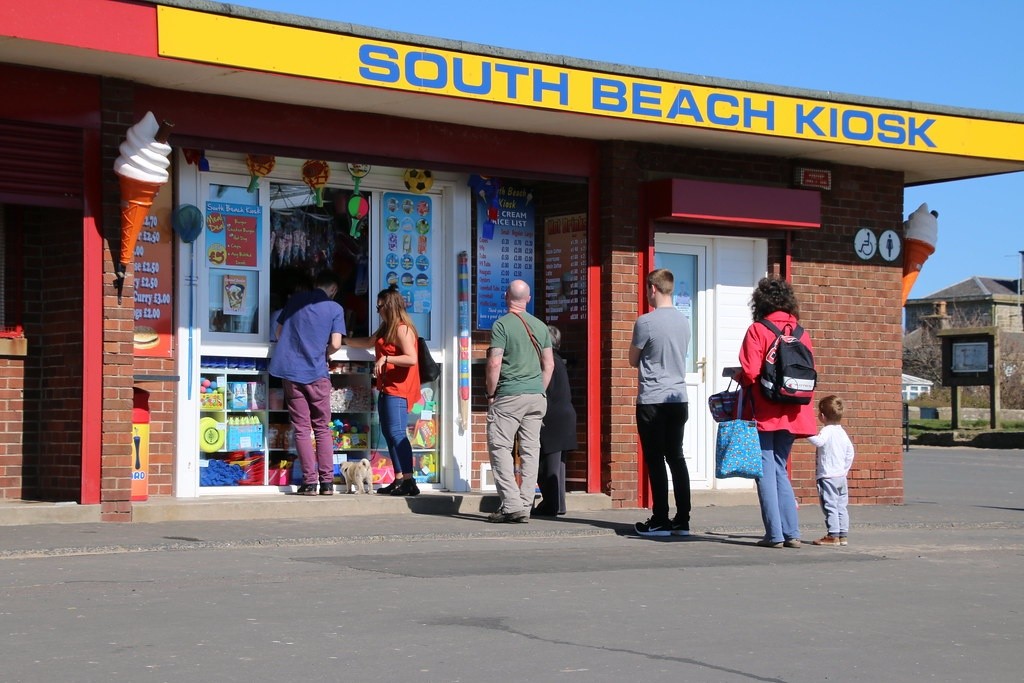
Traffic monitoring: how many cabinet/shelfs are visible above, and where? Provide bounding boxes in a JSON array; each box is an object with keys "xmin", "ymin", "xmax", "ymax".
[{"xmin": 201, "ymin": 359, "xmax": 444, "ymax": 493}]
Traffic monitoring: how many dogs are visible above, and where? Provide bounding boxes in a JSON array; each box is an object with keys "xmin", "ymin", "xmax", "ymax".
[{"xmin": 339, "ymin": 458, "xmax": 374, "ymax": 496}]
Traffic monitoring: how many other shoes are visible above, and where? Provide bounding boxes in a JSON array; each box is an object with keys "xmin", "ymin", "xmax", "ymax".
[
  {"xmin": 488, "ymin": 508, "xmax": 526, "ymax": 523},
  {"xmin": 813, "ymin": 535, "xmax": 840, "ymax": 546},
  {"xmin": 530, "ymin": 508, "xmax": 557, "ymax": 520},
  {"xmin": 511, "ymin": 510, "xmax": 529, "ymax": 523},
  {"xmin": 784, "ymin": 538, "xmax": 801, "ymax": 547},
  {"xmin": 757, "ymin": 539, "xmax": 783, "ymax": 548},
  {"xmin": 556, "ymin": 512, "xmax": 566, "ymax": 518},
  {"xmin": 319, "ymin": 482, "xmax": 333, "ymax": 494},
  {"xmin": 840, "ymin": 537, "xmax": 848, "ymax": 546},
  {"xmin": 297, "ymin": 484, "xmax": 317, "ymax": 496}
]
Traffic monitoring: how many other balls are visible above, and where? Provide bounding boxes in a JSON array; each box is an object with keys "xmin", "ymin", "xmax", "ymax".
[
  {"xmin": 200, "ymin": 378, "xmax": 224, "ymax": 394},
  {"xmin": 328, "ymin": 417, "xmax": 370, "ymax": 434}
]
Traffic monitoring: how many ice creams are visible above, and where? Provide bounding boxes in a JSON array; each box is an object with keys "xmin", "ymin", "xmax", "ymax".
[
  {"xmin": 901, "ymin": 202, "xmax": 938, "ymax": 307},
  {"xmin": 114, "ymin": 111, "xmax": 174, "ymax": 268}
]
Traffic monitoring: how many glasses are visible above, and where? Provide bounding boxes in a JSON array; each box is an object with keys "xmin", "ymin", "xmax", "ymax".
[{"xmin": 376, "ymin": 304, "xmax": 384, "ymax": 312}]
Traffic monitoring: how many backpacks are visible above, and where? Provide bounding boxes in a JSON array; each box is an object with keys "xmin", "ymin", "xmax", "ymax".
[{"xmin": 756, "ymin": 319, "xmax": 817, "ymax": 406}]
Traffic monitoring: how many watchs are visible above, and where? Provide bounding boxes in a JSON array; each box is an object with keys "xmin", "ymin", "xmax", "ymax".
[{"xmin": 484, "ymin": 393, "xmax": 495, "ymax": 399}]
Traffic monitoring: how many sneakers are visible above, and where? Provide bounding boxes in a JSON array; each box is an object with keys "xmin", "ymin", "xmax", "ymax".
[
  {"xmin": 389, "ymin": 479, "xmax": 420, "ymax": 497},
  {"xmin": 635, "ymin": 515, "xmax": 671, "ymax": 536},
  {"xmin": 670, "ymin": 518, "xmax": 689, "ymax": 536},
  {"xmin": 376, "ymin": 479, "xmax": 403, "ymax": 494}
]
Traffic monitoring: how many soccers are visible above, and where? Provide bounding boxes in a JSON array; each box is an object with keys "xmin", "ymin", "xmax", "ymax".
[{"xmin": 403, "ymin": 167, "xmax": 433, "ymax": 195}]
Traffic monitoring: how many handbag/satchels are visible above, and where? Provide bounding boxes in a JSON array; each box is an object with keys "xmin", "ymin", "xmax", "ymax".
[
  {"xmin": 708, "ymin": 368, "xmax": 742, "ymax": 423},
  {"xmin": 417, "ymin": 337, "xmax": 442, "ymax": 384},
  {"xmin": 715, "ymin": 377, "xmax": 764, "ymax": 479}
]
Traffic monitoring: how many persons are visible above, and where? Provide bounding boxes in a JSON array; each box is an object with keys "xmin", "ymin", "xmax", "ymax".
[
  {"xmin": 801, "ymin": 394, "xmax": 855, "ymax": 547},
  {"xmin": 722, "ymin": 274, "xmax": 819, "ymax": 548},
  {"xmin": 487, "ymin": 280, "xmax": 555, "ymax": 525},
  {"xmin": 269, "ymin": 305, "xmax": 285, "ymax": 342},
  {"xmin": 266, "ymin": 273, "xmax": 344, "ymax": 495},
  {"xmin": 530, "ymin": 324, "xmax": 577, "ymax": 517},
  {"xmin": 621, "ymin": 266, "xmax": 694, "ymax": 536},
  {"xmin": 337, "ymin": 287, "xmax": 420, "ymax": 496}
]
